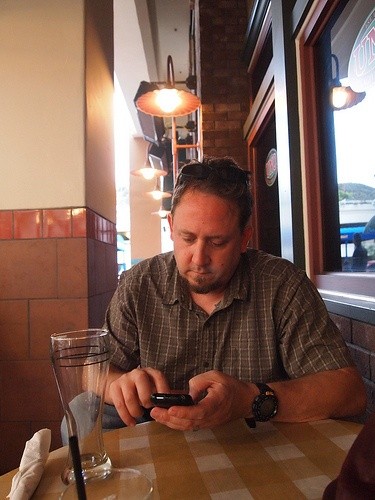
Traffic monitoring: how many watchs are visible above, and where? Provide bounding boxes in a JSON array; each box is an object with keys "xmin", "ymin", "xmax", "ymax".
[{"xmin": 245, "ymin": 383, "xmax": 278, "ymax": 428}]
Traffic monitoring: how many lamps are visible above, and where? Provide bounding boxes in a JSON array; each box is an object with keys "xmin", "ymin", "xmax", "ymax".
[
  {"xmin": 130, "ymin": 143, "xmax": 171, "ymax": 220},
  {"xmin": 332, "ymin": 54, "xmax": 367, "ymax": 114},
  {"xmin": 134, "ymin": 64, "xmax": 201, "ymax": 120}
]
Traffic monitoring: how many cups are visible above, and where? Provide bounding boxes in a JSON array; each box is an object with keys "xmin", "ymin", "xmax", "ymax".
[
  {"xmin": 49, "ymin": 328, "xmax": 116, "ymax": 490},
  {"xmin": 57, "ymin": 466, "xmax": 154, "ymax": 500}
]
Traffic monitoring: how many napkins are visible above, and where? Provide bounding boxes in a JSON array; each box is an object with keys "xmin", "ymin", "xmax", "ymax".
[{"xmin": 7, "ymin": 428, "xmax": 51, "ymax": 500}]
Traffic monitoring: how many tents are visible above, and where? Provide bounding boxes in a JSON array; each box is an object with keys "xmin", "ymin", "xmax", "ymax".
[{"xmin": 340, "ymin": 223, "xmax": 375, "ymax": 261}]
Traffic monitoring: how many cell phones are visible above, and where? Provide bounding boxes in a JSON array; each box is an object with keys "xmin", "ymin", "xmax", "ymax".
[{"xmin": 151, "ymin": 393, "xmax": 194, "ymax": 409}]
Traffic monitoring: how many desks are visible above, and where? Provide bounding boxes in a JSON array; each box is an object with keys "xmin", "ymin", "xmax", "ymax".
[{"xmin": 0, "ymin": 416, "xmax": 362, "ymax": 500}]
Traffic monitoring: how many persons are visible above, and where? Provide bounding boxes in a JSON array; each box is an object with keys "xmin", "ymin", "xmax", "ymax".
[
  {"xmin": 61, "ymin": 155, "xmax": 368, "ymax": 447},
  {"xmin": 353, "ymin": 234, "xmax": 368, "ymax": 272}
]
